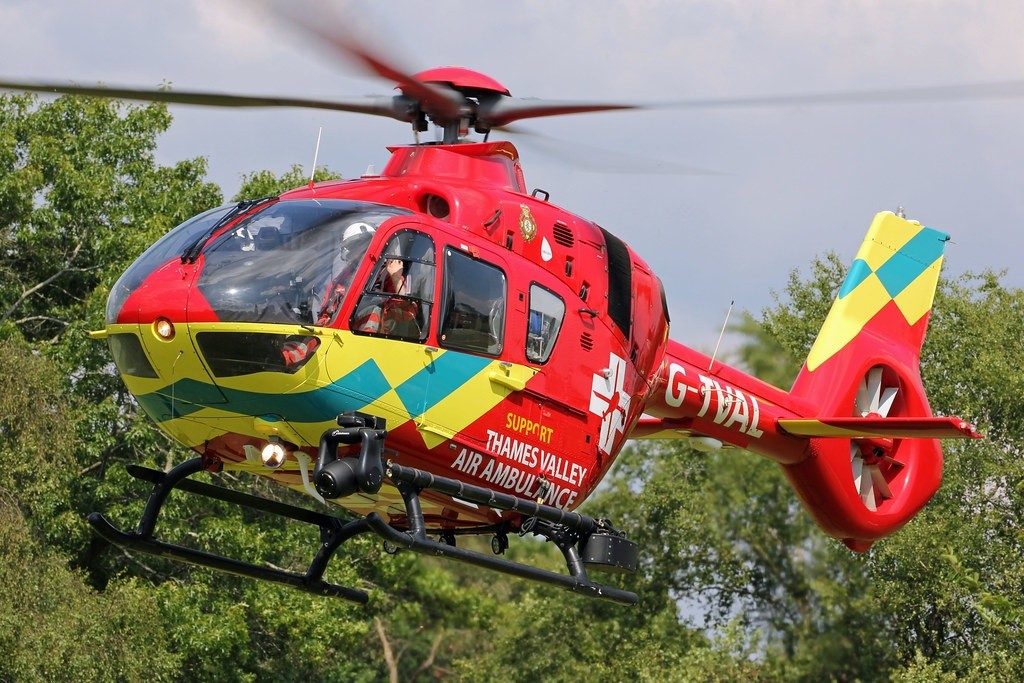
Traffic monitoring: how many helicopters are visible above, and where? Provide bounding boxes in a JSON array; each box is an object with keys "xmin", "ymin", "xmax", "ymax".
[{"xmin": 0, "ymin": 2, "xmax": 1024, "ymax": 604}]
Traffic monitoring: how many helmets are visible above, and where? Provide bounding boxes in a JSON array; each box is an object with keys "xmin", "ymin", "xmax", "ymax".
[{"xmin": 339, "ymin": 222, "xmax": 376, "ymax": 261}]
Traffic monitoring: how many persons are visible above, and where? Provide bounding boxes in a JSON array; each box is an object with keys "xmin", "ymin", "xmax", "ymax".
[{"xmin": 281, "ymin": 222, "xmax": 420, "ymax": 368}]
